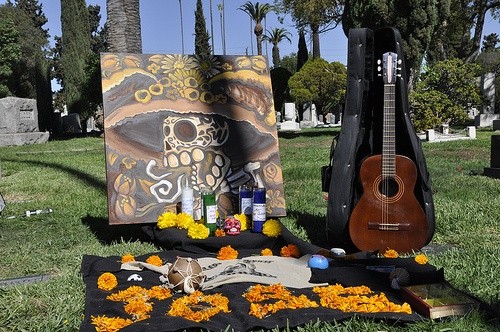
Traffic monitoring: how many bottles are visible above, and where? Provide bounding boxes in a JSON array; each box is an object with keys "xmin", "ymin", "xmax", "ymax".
[
  {"xmin": 181, "ymin": 178, "xmax": 193, "ymax": 213},
  {"xmin": 238, "ymin": 184, "xmax": 252, "ymax": 219},
  {"xmin": 252, "ymin": 187, "xmax": 267, "ymax": 232},
  {"xmin": 202, "ymin": 189, "xmax": 217, "ymax": 236}
]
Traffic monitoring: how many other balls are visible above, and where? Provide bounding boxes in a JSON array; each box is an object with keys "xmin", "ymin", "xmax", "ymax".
[{"xmin": 168, "ymin": 258, "xmax": 202, "ymax": 291}]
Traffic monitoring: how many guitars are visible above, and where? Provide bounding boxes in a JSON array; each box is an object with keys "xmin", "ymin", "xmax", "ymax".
[{"xmin": 348, "ymin": 51, "xmax": 428, "ymax": 254}]
